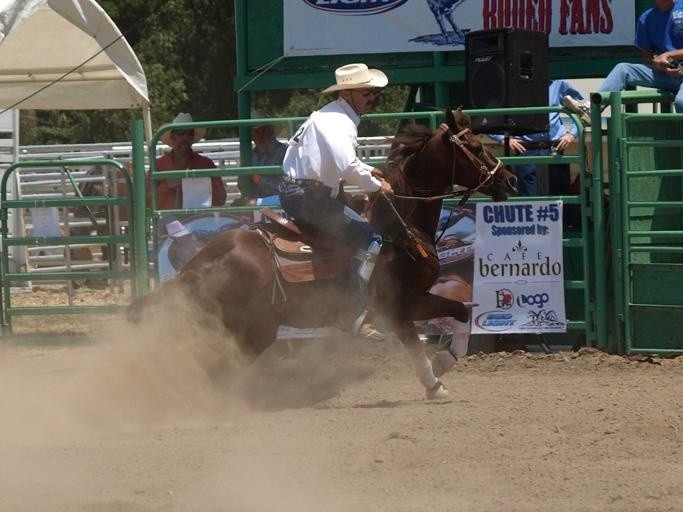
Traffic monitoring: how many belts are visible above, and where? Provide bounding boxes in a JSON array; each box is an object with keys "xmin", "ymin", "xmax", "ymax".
[{"xmin": 521, "ymin": 141, "xmax": 561, "ymax": 150}]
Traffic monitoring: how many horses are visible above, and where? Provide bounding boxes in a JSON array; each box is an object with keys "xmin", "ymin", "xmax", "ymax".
[{"xmin": 128, "ymin": 104, "xmax": 518, "ymax": 400}]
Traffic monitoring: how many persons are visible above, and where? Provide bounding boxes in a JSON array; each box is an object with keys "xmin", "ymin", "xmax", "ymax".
[
  {"xmin": 563, "ymin": 0, "xmax": 683, "ymax": 127},
  {"xmin": 487, "ymin": 79, "xmax": 583, "ymax": 234},
  {"xmin": 278, "ymin": 62, "xmax": 394, "ymax": 343},
  {"xmin": 232, "ymin": 122, "xmax": 287, "ymax": 207},
  {"xmin": 146, "ymin": 113, "xmax": 226, "ymax": 279}
]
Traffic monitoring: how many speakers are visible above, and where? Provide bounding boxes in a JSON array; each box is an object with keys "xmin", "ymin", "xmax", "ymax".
[{"xmin": 464, "ymin": 27, "xmax": 550, "ymax": 135}]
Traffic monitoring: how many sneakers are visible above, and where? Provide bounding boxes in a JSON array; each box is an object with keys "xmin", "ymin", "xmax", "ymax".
[
  {"xmin": 337, "ymin": 307, "xmax": 386, "ymax": 342},
  {"xmin": 562, "ymin": 92, "xmax": 593, "ymax": 127}
]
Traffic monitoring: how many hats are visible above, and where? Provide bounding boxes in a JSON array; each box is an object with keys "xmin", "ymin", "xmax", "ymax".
[
  {"xmin": 158, "ymin": 111, "xmax": 209, "ymax": 148},
  {"xmin": 320, "ymin": 62, "xmax": 390, "ymax": 96},
  {"xmin": 250, "ymin": 109, "xmax": 283, "ymax": 138}
]
